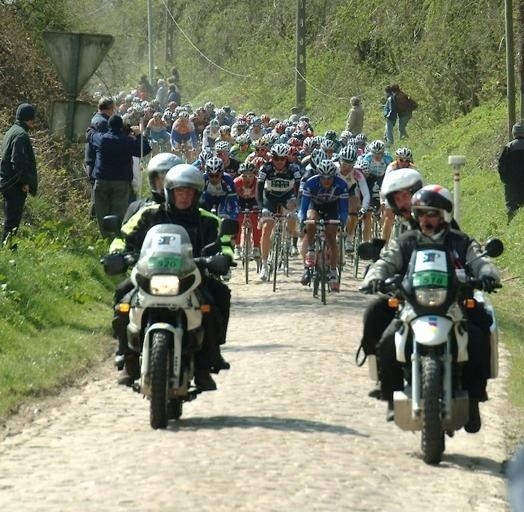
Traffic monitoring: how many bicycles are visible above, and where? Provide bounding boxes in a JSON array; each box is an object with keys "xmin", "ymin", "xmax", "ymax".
[
  {"xmin": 148, "ymin": 140, "xmax": 411, "ymax": 292},
  {"xmin": 299, "ymin": 208, "xmax": 346, "ymax": 305}
]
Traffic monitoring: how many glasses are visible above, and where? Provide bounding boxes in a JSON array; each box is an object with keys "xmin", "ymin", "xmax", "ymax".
[{"xmin": 414, "ymin": 209, "xmax": 439, "ymax": 218}]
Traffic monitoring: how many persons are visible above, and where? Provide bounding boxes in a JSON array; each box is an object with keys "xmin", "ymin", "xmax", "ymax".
[
  {"xmin": 392, "ymin": 84, "xmax": 412, "ymax": 140},
  {"xmin": 194, "ymin": 101, "xmax": 302, "ymax": 284},
  {"xmin": 115, "ymin": 67, "xmax": 199, "ymax": 164},
  {"xmin": 384, "ymin": 85, "xmax": 397, "ymax": 144},
  {"xmin": 104, "ymin": 163, "xmax": 221, "ymax": 390},
  {"xmin": 498, "ymin": 122, "xmax": 524, "ymax": 223},
  {"xmin": 87, "ymin": 115, "xmax": 152, "ymax": 239},
  {"xmin": 362, "ymin": 169, "xmax": 461, "ymax": 359},
  {"xmin": 302, "ymin": 117, "xmax": 412, "ymax": 291},
  {"xmin": 87, "ymin": 97, "xmax": 114, "ymax": 223},
  {"xmin": 110, "ymin": 153, "xmax": 232, "ymax": 370},
  {"xmin": 0, "ymin": 102, "xmax": 37, "ymax": 248},
  {"xmin": 364, "ymin": 185, "xmax": 501, "ymax": 434}
]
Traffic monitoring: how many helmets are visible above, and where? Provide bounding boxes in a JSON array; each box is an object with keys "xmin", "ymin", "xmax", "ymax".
[
  {"xmin": 162, "ymin": 162, "xmax": 205, "ymax": 213},
  {"xmin": 148, "ymin": 152, "xmax": 185, "ymax": 202},
  {"xmin": 92, "ymin": 91, "xmax": 192, "ymax": 121},
  {"xmin": 411, "ymin": 185, "xmax": 454, "ymax": 225},
  {"xmin": 379, "ymin": 168, "xmax": 423, "ymax": 209},
  {"xmin": 305, "ymin": 131, "xmax": 414, "ymax": 175},
  {"xmin": 197, "ymin": 102, "xmax": 309, "ymax": 173}
]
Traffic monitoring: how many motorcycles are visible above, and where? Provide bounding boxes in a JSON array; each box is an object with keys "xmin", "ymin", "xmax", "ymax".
[
  {"xmin": 102, "ymin": 213, "xmax": 239, "ymax": 431},
  {"xmin": 354, "ymin": 237, "xmax": 504, "ymax": 466}
]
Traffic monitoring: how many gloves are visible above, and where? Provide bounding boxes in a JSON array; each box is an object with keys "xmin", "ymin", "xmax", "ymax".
[
  {"xmin": 103, "ymin": 253, "xmax": 127, "ymax": 276},
  {"xmin": 207, "ymin": 253, "xmax": 229, "ymax": 277},
  {"xmin": 367, "ymin": 278, "xmax": 383, "ymax": 290},
  {"xmin": 481, "ymin": 275, "xmax": 497, "ymax": 295}
]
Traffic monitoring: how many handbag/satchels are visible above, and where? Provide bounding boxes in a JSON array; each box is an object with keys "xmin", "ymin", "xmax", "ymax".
[{"xmin": 406, "ymin": 97, "xmax": 417, "ymax": 111}]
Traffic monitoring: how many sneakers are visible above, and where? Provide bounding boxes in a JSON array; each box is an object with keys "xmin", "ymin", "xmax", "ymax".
[
  {"xmin": 194, "ymin": 373, "xmax": 216, "ymax": 391},
  {"xmin": 233, "ymin": 245, "xmax": 241, "ymax": 260},
  {"xmin": 113, "ymin": 354, "xmax": 124, "ymax": 366},
  {"xmin": 465, "ymin": 396, "xmax": 481, "ymax": 434},
  {"xmin": 118, "ymin": 371, "xmax": 131, "ymax": 383},
  {"xmin": 258, "ymin": 249, "xmax": 340, "ymax": 292},
  {"xmin": 345, "ymin": 237, "xmax": 354, "ymax": 253},
  {"xmin": 213, "ymin": 352, "xmax": 230, "ymax": 371}
]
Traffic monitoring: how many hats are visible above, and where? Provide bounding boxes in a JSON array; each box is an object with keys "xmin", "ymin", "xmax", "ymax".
[
  {"xmin": 510, "ymin": 120, "xmax": 524, "ymax": 135},
  {"xmin": 15, "ymin": 104, "xmax": 34, "ymax": 121}
]
